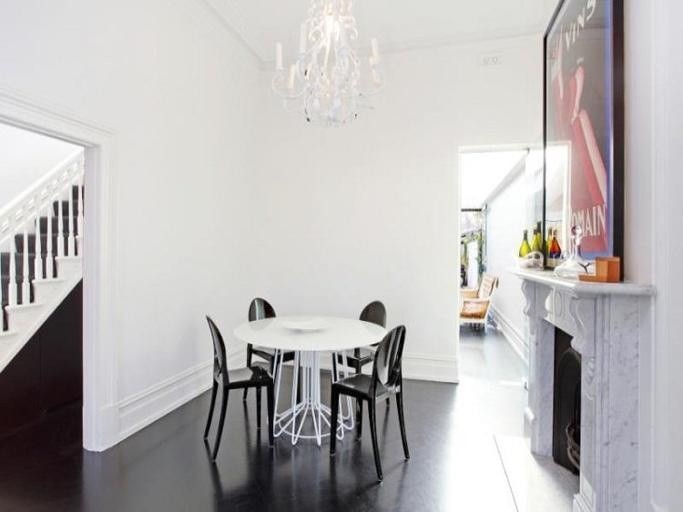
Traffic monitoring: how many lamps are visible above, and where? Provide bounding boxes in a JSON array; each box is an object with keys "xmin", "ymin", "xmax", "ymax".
[{"xmin": 270, "ymin": 0, "xmax": 389, "ymax": 127}]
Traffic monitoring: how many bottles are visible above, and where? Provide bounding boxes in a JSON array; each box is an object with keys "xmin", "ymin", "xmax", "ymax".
[
  {"xmin": 518, "ymin": 221, "xmax": 561, "ymax": 259},
  {"xmin": 553, "ymin": 225, "xmax": 593, "ymax": 282}
]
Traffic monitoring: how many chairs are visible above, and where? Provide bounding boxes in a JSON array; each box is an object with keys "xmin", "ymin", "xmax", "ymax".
[
  {"xmin": 200, "ymin": 315, "xmax": 278, "ymax": 461},
  {"xmin": 246, "ymin": 298, "xmax": 302, "ymax": 422},
  {"xmin": 461, "ymin": 272, "xmax": 498, "ymax": 331},
  {"xmin": 333, "ymin": 300, "xmax": 391, "ymax": 440},
  {"xmin": 330, "ymin": 324, "xmax": 410, "ymax": 481}
]
